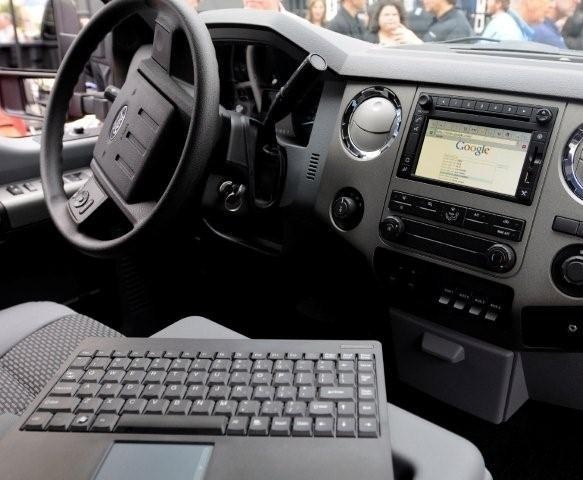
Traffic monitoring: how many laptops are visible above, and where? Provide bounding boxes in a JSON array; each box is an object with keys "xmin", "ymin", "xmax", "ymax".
[{"xmin": 0, "ymin": 335, "xmax": 394, "ymax": 480}]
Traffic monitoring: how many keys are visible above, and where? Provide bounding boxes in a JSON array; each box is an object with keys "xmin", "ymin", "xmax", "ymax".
[{"xmin": 223, "ymin": 184, "xmax": 246, "ymax": 202}]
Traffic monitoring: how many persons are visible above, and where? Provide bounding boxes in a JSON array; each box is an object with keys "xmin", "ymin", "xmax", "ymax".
[
  {"xmin": 242, "ymin": 1, "xmax": 582, "ymax": 50},
  {"xmin": 0, "ymin": 12, "xmax": 27, "ymax": 45}
]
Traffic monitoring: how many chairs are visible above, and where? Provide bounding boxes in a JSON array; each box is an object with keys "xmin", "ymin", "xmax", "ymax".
[{"xmin": 0, "ymin": 299, "xmax": 128, "ymax": 416}]
